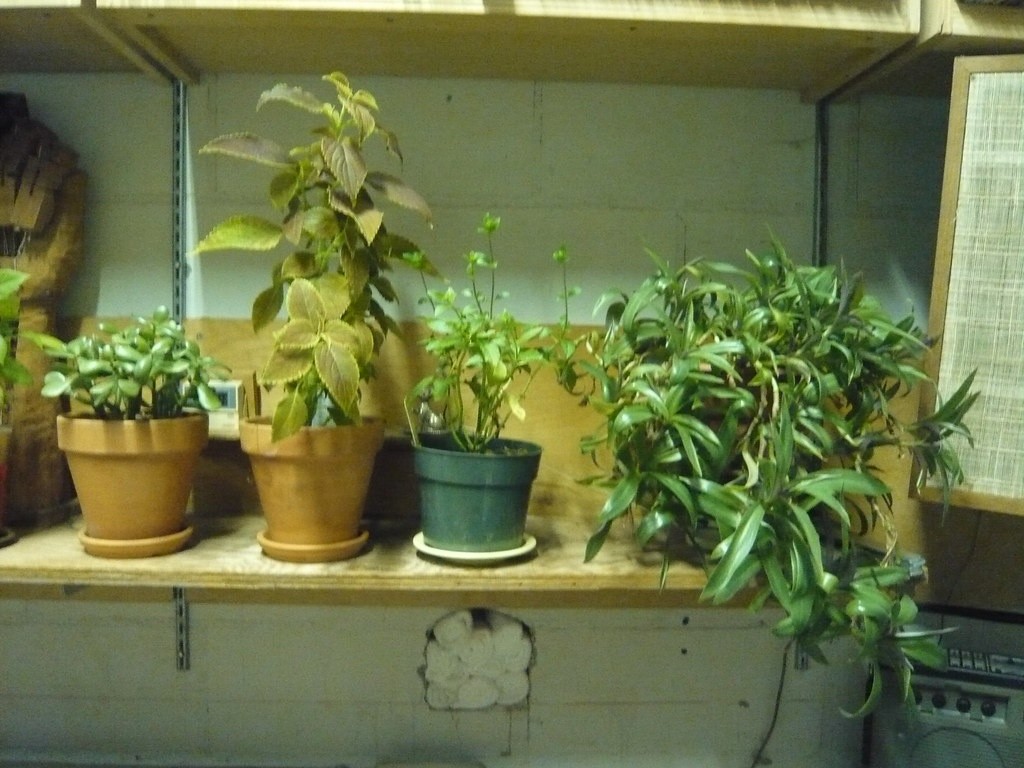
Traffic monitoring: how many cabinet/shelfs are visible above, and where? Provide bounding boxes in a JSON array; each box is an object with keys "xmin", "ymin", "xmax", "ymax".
[
  {"xmin": 0, "ymin": 580, "xmax": 871, "ymax": 768},
  {"xmin": 1, "ymin": 0, "xmax": 1024, "ymax": 105}
]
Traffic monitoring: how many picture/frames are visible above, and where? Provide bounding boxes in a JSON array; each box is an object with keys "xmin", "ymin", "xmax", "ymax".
[{"xmin": 907, "ymin": 53, "xmax": 1024, "ymax": 516}]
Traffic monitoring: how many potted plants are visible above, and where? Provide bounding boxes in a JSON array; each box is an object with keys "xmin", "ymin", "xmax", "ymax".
[
  {"xmin": 573, "ymin": 221, "xmax": 981, "ymax": 723},
  {"xmin": 41, "ymin": 306, "xmax": 231, "ymax": 557},
  {"xmin": 184, "ymin": 70, "xmax": 450, "ymax": 564},
  {"xmin": 0, "ymin": 268, "xmax": 64, "ymax": 541},
  {"xmin": 402, "ymin": 212, "xmax": 579, "ymax": 566}
]
